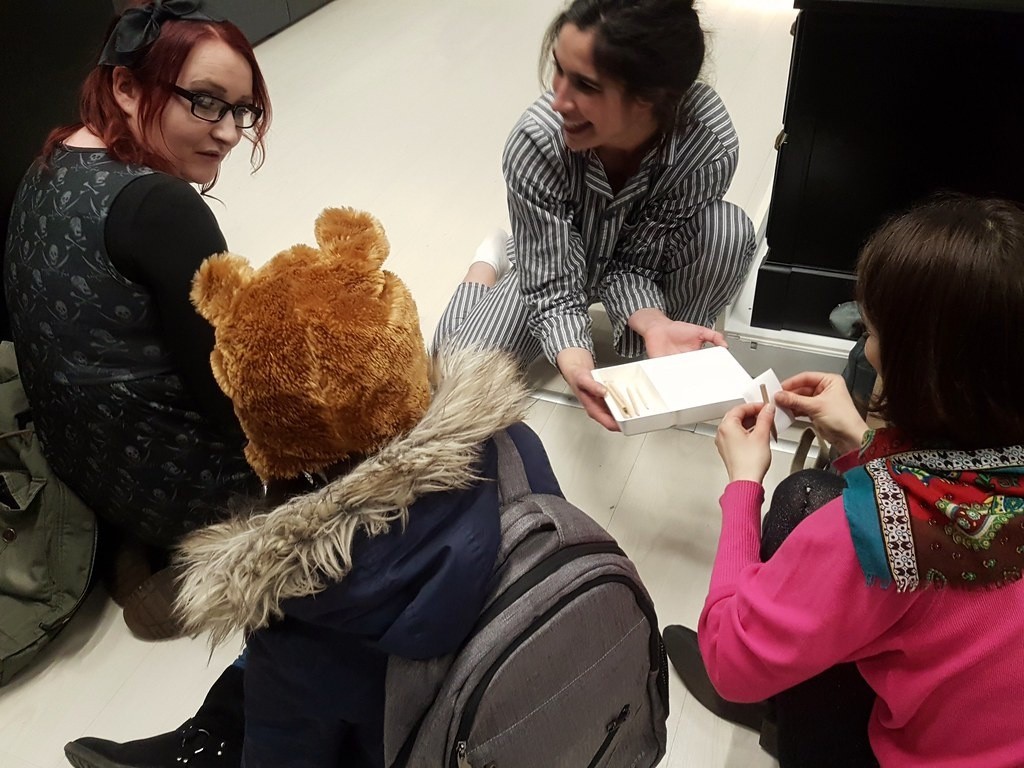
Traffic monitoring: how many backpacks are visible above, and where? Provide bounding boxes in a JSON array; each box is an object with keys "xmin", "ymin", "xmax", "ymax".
[{"xmin": 386, "ymin": 428, "xmax": 669, "ymax": 768}]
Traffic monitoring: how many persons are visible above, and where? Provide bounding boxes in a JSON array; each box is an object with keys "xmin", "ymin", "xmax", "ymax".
[
  {"xmin": 662, "ymin": 195, "xmax": 1024, "ymax": 768},
  {"xmin": 430, "ymin": 0, "xmax": 757, "ymax": 432},
  {"xmin": 62, "ymin": 203, "xmax": 670, "ymax": 768},
  {"xmin": 4, "ymin": 1, "xmax": 272, "ymax": 641}
]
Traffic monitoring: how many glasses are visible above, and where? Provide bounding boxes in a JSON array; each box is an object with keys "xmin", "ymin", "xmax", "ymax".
[{"xmin": 159, "ymin": 80, "xmax": 264, "ymax": 129}]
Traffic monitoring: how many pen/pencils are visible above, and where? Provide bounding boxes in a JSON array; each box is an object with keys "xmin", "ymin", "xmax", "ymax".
[
  {"xmin": 760, "ymin": 384, "xmax": 778, "ymax": 443},
  {"xmin": 605, "ymin": 382, "xmax": 648, "ymax": 421}
]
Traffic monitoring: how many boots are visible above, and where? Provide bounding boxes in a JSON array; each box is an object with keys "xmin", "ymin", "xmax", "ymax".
[{"xmin": 64, "ymin": 664, "xmax": 245, "ymax": 768}]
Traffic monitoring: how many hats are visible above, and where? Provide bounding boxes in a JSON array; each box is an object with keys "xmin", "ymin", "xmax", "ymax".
[{"xmin": 189, "ymin": 208, "xmax": 434, "ymax": 485}]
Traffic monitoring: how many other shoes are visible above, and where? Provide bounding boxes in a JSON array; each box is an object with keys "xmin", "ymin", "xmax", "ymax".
[
  {"xmin": 661, "ymin": 624, "xmax": 782, "ymax": 761},
  {"xmin": 100, "ymin": 538, "xmax": 200, "ymax": 641}
]
